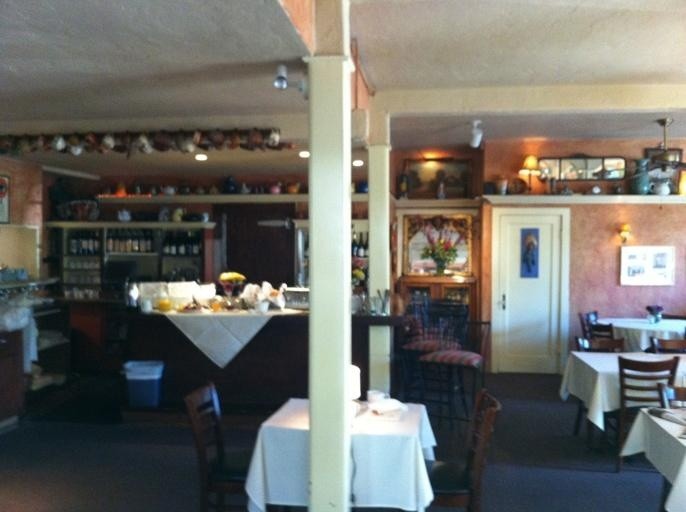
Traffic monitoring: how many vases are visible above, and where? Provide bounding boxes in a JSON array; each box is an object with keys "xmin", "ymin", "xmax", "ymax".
[{"xmin": 435, "ymin": 257, "xmax": 447, "ymax": 275}]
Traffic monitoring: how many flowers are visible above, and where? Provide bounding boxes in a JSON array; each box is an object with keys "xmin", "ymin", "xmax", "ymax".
[
  {"xmin": 350, "ymin": 259, "xmax": 369, "ymax": 290},
  {"xmin": 217, "ymin": 270, "xmax": 248, "ymax": 296},
  {"xmin": 420, "ymin": 227, "xmax": 468, "ymax": 263}
]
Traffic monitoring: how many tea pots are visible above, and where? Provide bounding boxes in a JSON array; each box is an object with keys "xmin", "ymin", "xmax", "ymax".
[
  {"xmin": 116, "ymin": 207, "xmax": 131, "ymax": 222},
  {"xmin": 649, "ymin": 181, "xmax": 671, "ymax": 195},
  {"xmin": 159, "ymin": 183, "xmax": 179, "ymax": 196}
]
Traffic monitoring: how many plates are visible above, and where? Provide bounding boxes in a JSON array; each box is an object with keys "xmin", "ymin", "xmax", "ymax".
[{"xmin": 508, "ymin": 178, "xmax": 527, "ymax": 194}]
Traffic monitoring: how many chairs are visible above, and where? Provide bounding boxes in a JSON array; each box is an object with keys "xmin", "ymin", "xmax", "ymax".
[
  {"xmin": 422, "ymin": 321, "xmax": 491, "ymax": 439},
  {"xmin": 397, "ymin": 295, "xmax": 463, "ymax": 355},
  {"xmin": 557, "ymin": 306, "xmax": 685, "ymax": 512},
  {"xmin": 424, "ymin": 389, "xmax": 503, "ymax": 512},
  {"xmin": 180, "ymin": 381, "xmax": 251, "ymax": 512},
  {"xmin": 402, "ymin": 303, "xmax": 471, "ymax": 431}
]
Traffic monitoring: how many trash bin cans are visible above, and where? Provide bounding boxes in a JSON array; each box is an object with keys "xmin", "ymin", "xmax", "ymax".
[{"xmin": 123, "ymin": 361, "xmax": 164, "ymax": 410}]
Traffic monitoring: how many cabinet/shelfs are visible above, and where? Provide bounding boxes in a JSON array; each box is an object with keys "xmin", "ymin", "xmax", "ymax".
[
  {"xmin": 399, "ymin": 276, "xmax": 481, "ymax": 343},
  {"xmin": 295, "ymin": 220, "xmax": 370, "ymax": 298},
  {"xmin": 0, "ymin": 320, "xmax": 28, "ymax": 434},
  {"xmin": 46, "ymin": 221, "xmax": 214, "ymax": 308},
  {"xmin": 68, "ymin": 303, "xmax": 103, "ymax": 351},
  {"xmin": 0, "ymin": 274, "xmax": 73, "ymax": 405}
]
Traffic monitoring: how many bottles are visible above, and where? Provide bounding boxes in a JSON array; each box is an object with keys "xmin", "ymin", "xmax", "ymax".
[
  {"xmin": 165, "ymin": 267, "xmax": 201, "ymax": 282},
  {"xmin": 69, "ymin": 228, "xmax": 200, "ymax": 254},
  {"xmin": 370, "ymin": 299, "xmax": 376, "ymax": 317},
  {"xmin": 350, "ymin": 231, "xmax": 370, "ymax": 257}
]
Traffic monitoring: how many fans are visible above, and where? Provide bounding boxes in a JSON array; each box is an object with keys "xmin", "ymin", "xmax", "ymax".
[{"xmin": 596, "ymin": 117, "xmax": 686, "ymax": 187}]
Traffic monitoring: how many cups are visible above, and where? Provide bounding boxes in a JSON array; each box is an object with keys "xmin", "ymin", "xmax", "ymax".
[
  {"xmin": 498, "ymin": 179, "xmax": 508, "ymax": 195},
  {"xmin": 136, "ymin": 293, "xmax": 243, "ymax": 315},
  {"xmin": 376, "ymin": 297, "xmax": 388, "ymax": 317},
  {"xmin": 69, "ymin": 260, "xmax": 100, "ymax": 271},
  {"xmin": 366, "ymin": 390, "xmax": 389, "ymax": 404},
  {"xmin": 63, "ymin": 287, "xmax": 99, "ymax": 301},
  {"xmin": 254, "ymin": 301, "xmax": 269, "ymax": 313},
  {"xmin": 67, "ymin": 274, "xmax": 99, "ymax": 285}
]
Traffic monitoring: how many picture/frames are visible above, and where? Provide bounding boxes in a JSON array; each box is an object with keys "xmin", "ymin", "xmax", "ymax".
[{"xmin": 402, "ymin": 156, "xmax": 474, "ymax": 199}]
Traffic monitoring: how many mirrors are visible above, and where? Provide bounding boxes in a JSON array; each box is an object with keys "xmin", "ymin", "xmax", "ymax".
[{"xmin": 403, "ymin": 212, "xmax": 473, "ymax": 278}]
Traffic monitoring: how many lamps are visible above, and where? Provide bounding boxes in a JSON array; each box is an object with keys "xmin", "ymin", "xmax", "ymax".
[
  {"xmin": 515, "ymin": 154, "xmax": 542, "ymax": 194},
  {"xmin": 616, "ymin": 222, "xmax": 632, "ymax": 244}
]
